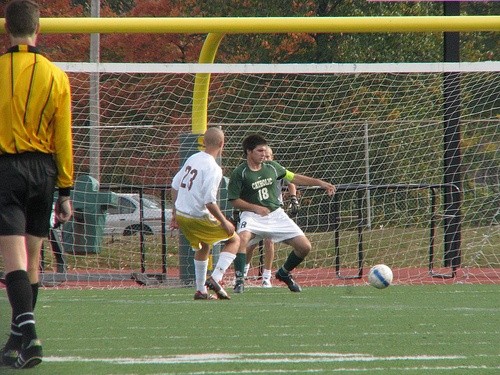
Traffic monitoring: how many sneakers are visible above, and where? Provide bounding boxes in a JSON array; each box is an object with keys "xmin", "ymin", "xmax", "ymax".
[
  {"xmin": 204, "ymin": 277, "xmax": 231, "ymax": 299},
  {"xmin": 233, "ymin": 279, "xmax": 244, "ymax": 293},
  {"xmin": 194, "ymin": 291, "xmax": 218, "ymax": 300},
  {"xmin": 13, "ymin": 339, "xmax": 42, "ymax": 369},
  {"xmin": 262, "ymin": 280, "xmax": 272, "ymax": 288},
  {"xmin": 0, "ymin": 349, "xmax": 19, "ymax": 364},
  {"xmin": 276, "ymin": 270, "xmax": 302, "ymax": 292}
]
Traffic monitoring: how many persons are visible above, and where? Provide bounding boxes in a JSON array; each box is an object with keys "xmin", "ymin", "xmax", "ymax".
[
  {"xmin": 227, "ymin": 133, "xmax": 337, "ymax": 293},
  {"xmin": 233, "ymin": 146, "xmax": 303, "ymax": 289},
  {"xmin": 167, "ymin": 126, "xmax": 241, "ymax": 300},
  {"xmin": 0, "ymin": 0, "xmax": 76, "ymax": 369}
]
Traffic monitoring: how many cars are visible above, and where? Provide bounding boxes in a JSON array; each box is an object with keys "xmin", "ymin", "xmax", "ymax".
[{"xmin": 104, "ymin": 193, "xmax": 173, "ymax": 235}]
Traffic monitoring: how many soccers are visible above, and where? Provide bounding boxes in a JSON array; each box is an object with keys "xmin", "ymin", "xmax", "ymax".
[{"xmin": 367, "ymin": 264, "xmax": 393, "ymax": 289}]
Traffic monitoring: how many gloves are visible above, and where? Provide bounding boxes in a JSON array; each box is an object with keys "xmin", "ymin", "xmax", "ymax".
[
  {"xmin": 289, "ymin": 195, "xmax": 301, "ymax": 214},
  {"xmin": 234, "ymin": 211, "xmax": 240, "ymax": 222}
]
